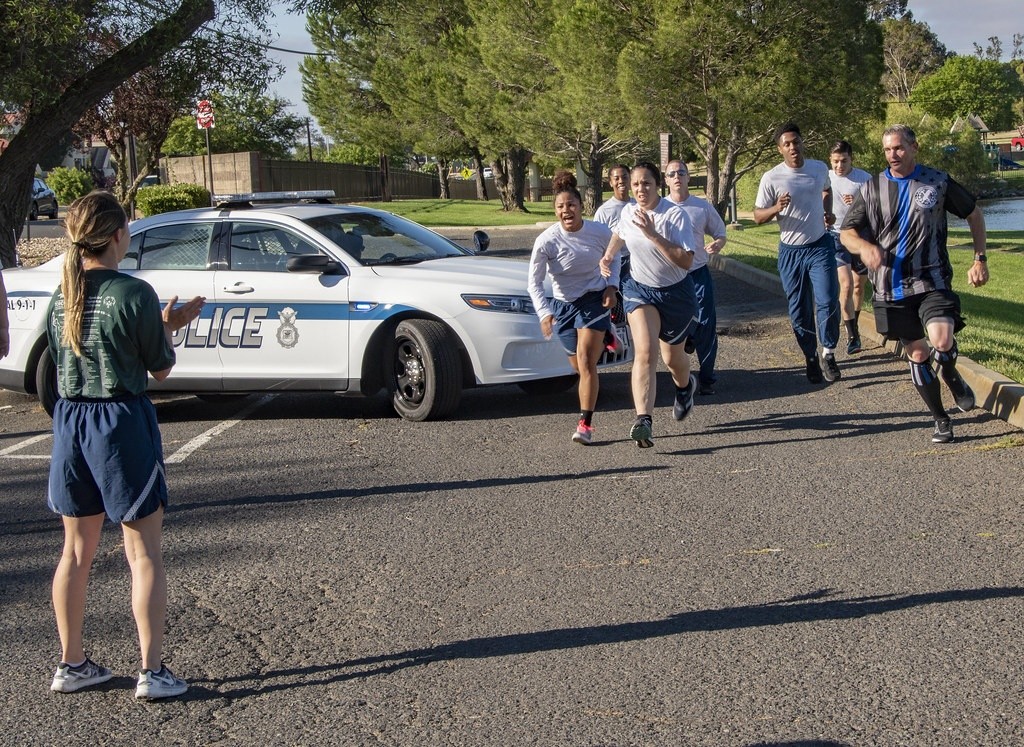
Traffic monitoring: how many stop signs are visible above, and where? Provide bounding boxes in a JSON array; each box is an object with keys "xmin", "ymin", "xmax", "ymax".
[{"xmin": 197, "ymin": 101, "xmax": 215, "ymax": 128}]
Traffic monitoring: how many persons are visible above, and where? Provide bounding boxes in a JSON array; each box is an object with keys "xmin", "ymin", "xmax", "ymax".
[
  {"xmin": 594, "ymin": 161, "xmax": 697, "ymax": 449},
  {"xmin": 663, "ymin": 159, "xmax": 726, "ymax": 395},
  {"xmin": 840, "ymin": 124, "xmax": 990, "ymax": 444},
  {"xmin": 0, "ymin": 258, "xmax": 10, "ymax": 360},
  {"xmin": 753, "ymin": 124, "xmax": 841, "ymax": 383},
  {"xmin": 527, "ymin": 170, "xmax": 625, "ymax": 445},
  {"xmin": 823, "ymin": 141, "xmax": 873, "ymax": 353},
  {"xmin": 47, "ymin": 189, "xmax": 206, "ymax": 698}
]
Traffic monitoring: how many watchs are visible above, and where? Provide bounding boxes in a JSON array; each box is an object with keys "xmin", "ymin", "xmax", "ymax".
[{"xmin": 974, "ymin": 255, "xmax": 987, "ymax": 262}]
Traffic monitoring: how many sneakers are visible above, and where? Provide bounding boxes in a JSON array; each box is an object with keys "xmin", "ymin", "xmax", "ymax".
[
  {"xmin": 941, "ymin": 367, "xmax": 976, "ymax": 413},
  {"xmin": 606, "ymin": 322, "xmax": 626, "ymax": 355},
  {"xmin": 672, "ymin": 374, "xmax": 697, "ymax": 422},
  {"xmin": 630, "ymin": 416, "xmax": 654, "ymax": 448},
  {"xmin": 50, "ymin": 649, "xmax": 112, "ymax": 694},
  {"xmin": 806, "ymin": 350, "xmax": 822, "ymax": 384},
  {"xmin": 572, "ymin": 418, "xmax": 594, "ymax": 445},
  {"xmin": 932, "ymin": 416, "xmax": 954, "ymax": 444},
  {"xmin": 819, "ymin": 352, "xmax": 841, "ymax": 382},
  {"xmin": 135, "ymin": 657, "xmax": 188, "ymax": 699}
]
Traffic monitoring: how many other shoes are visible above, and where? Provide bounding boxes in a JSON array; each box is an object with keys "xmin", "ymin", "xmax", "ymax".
[
  {"xmin": 847, "ymin": 334, "xmax": 861, "ymax": 354},
  {"xmin": 699, "ymin": 377, "xmax": 715, "ymax": 395},
  {"xmin": 684, "ymin": 335, "xmax": 696, "ymax": 354}
]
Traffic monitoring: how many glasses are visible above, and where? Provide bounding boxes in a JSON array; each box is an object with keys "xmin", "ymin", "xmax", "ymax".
[{"xmin": 665, "ymin": 169, "xmax": 688, "ymax": 178}]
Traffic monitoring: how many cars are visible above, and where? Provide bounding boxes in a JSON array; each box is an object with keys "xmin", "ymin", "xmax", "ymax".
[
  {"xmin": 0, "ymin": 190, "xmax": 634, "ymax": 421},
  {"xmin": 27, "ymin": 178, "xmax": 58, "ymax": 221},
  {"xmin": 483, "ymin": 168, "xmax": 493, "ymax": 178}
]
